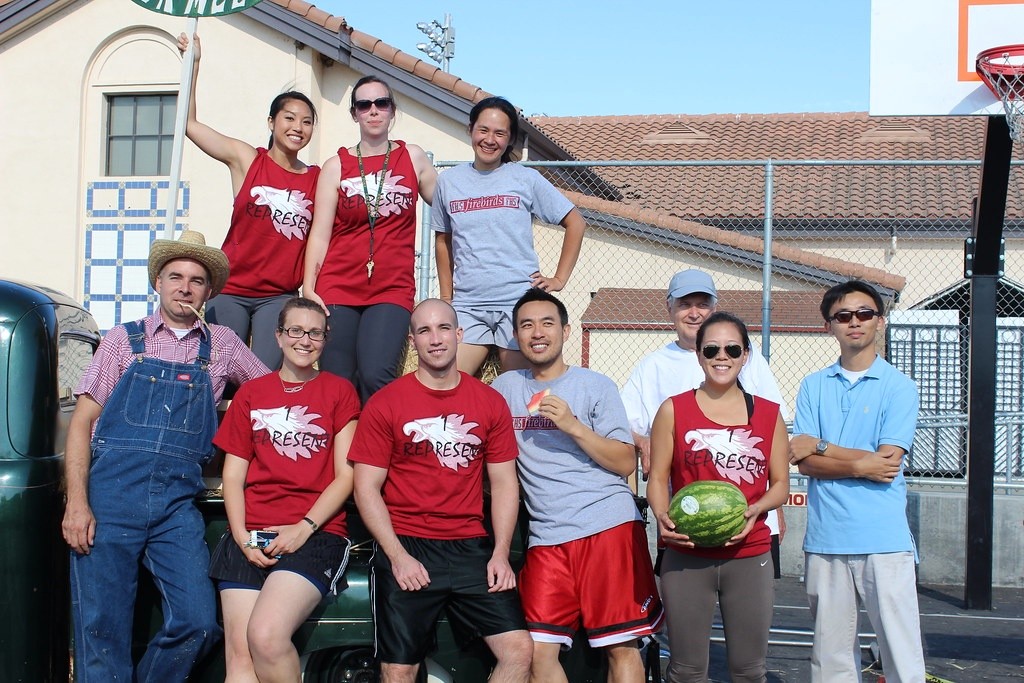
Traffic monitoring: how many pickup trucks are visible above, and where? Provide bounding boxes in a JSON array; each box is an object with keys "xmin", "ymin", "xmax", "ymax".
[{"xmin": 0, "ymin": 281, "xmax": 661, "ymax": 683}]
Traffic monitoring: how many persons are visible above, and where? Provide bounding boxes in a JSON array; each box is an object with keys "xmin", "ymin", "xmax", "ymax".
[
  {"xmin": 489, "ymin": 288, "xmax": 665, "ymax": 683},
  {"xmin": 301, "ymin": 75, "xmax": 439, "ymax": 407},
  {"xmin": 430, "ymin": 96, "xmax": 587, "ymax": 377},
  {"xmin": 176, "ymin": 31, "xmax": 322, "ymax": 401},
  {"xmin": 345, "ymin": 299, "xmax": 534, "ymax": 683},
  {"xmin": 208, "ymin": 298, "xmax": 361, "ymax": 683},
  {"xmin": 645, "ymin": 311, "xmax": 791, "ymax": 683},
  {"xmin": 787, "ymin": 280, "xmax": 926, "ymax": 683},
  {"xmin": 62, "ymin": 230, "xmax": 272, "ymax": 683},
  {"xmin": 622, "ymin": 270, "xmax": 788, "ymax": 631}
]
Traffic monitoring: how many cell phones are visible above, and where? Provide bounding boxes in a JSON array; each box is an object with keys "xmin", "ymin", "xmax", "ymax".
[{"xmin": 249, "ymin": 529, "xmax": 278, "ymax": 548}]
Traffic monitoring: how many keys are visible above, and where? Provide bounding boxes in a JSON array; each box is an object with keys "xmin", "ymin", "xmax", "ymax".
[{"xmin": 366, "ymin": 261, "xmax": 374, "ymax": 278}]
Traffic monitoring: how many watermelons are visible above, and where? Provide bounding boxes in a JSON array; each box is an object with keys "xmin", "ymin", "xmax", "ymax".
[{"xmin": 668, "ymin": 481, "xmax": 749, "ymax": 548}]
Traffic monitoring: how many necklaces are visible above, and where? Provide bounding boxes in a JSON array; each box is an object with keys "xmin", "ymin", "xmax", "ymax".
[{"xmin": 279, "ymin": 372, "xmax": 317, "ymax": 393}]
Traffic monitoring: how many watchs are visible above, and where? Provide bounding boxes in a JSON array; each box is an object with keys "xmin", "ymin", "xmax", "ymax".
[
  {"xmin": 303, "ymin": 515, "xmax": 318, "ymax": 531},
  {"xmin": 816, "ymin": 439, "xmax": 830, "ymax": 454}
]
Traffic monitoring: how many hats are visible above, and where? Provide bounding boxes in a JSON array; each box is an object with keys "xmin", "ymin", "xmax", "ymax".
[
  {"xmin": 147, "ymin": 229, "xmax": 230, "ymax": 299},
  {"xmin": 666, "ymin": 270, "xmax": 718, "ymax": 299}
]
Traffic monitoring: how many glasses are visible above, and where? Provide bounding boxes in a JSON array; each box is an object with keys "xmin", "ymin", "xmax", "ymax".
[
  {"xmin": 279, "ymin": 325, "xmax": 327, "ymax": 342},
  {"xmin": 700, "ymin": 344, "xmax": 745, "ymax": 359},
  {"xmin": 353, "ymin": 97, "xmax": 393, "ymax": 113},
  {"xmin": 828, "ymin": 309, "xmax": 881, "ymax": 324}
]
